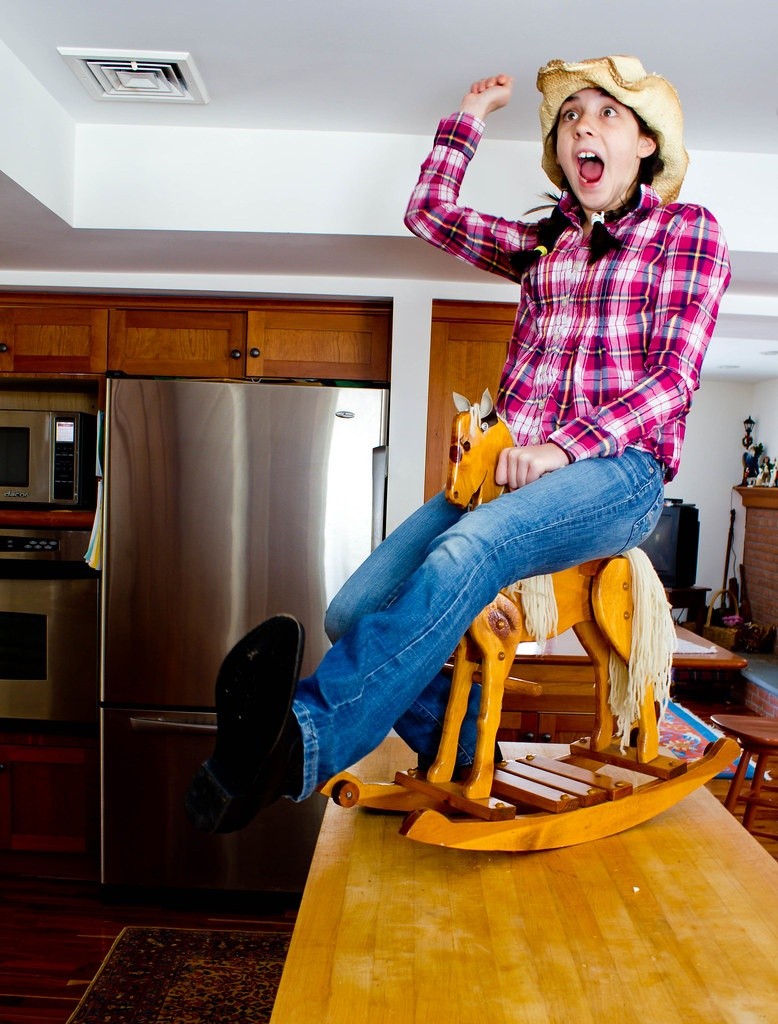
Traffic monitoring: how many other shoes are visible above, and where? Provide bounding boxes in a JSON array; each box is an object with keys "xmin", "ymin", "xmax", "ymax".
[
  {"xmin": 361, "ymin": 746, "xmax": 505, "ymax": 816},
  {"xmin": 183, "ymin": 615, "xmax": 305, "ymax": 832}
]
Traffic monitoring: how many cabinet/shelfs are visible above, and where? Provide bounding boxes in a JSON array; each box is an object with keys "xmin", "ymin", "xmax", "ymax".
[{"xmin": 0, "ymin": 301, "xmax": 392, "ymax": 381}]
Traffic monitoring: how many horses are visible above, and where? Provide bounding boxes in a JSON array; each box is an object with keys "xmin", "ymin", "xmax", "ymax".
[{"xmin": 424, "ymin": 389, "xmax": 676, "ymax": 798}]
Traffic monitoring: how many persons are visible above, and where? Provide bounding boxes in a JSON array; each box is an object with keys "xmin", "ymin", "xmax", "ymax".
[{"xmin": 183, "ymin": 54, "xmax": 732, "ymax": 834}]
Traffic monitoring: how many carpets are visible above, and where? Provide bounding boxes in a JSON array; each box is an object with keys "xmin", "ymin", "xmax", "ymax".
[{"xmin": 66, "ymin": 925, "xmax": 293, "ymax": 1024}]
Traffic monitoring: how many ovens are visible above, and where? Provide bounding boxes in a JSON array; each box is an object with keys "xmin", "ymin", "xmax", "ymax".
[{"xmin": 0, "ymin": 527, "xmax": 99, "ymax": 719}]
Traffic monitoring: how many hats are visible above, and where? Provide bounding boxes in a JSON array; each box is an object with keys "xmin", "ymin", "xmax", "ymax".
[{"xmin": 538, "ymin": 56, "xmax": 689, "ymax": 205}]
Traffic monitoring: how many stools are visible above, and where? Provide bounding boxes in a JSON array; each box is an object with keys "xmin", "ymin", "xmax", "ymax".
[{"xmin": 710, "ymin": 715, "xmax": 778, "ymax": 843}]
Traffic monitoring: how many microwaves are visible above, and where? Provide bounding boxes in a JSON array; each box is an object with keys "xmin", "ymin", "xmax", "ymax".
[{"xmin": 0, "ymin": 408, "xmax": 95, "ymax": 507}]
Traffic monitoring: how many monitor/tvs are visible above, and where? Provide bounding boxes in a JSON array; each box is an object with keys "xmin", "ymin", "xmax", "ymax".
[{"xmin": 637, "ymin": 505, "xmax": 700, "ymax": 588}]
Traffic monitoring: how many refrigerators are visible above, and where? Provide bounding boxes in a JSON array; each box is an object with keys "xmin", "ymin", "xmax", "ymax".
[{"xmin": 100, "ymin": 374, "xmax": 391, "ymax": 894}]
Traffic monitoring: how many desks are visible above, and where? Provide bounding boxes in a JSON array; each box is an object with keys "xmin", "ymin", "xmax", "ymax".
[
  {"xmin": 270, "ymin": 736, "xmax": 777, "ymax": 1024},
  {"xmin": 664, "ymin": 585, "xmax": 712, "ymax": 634},
  {"xmin": 447, "ymin": 623, "xmax": 748, "ymax": 744}
]
